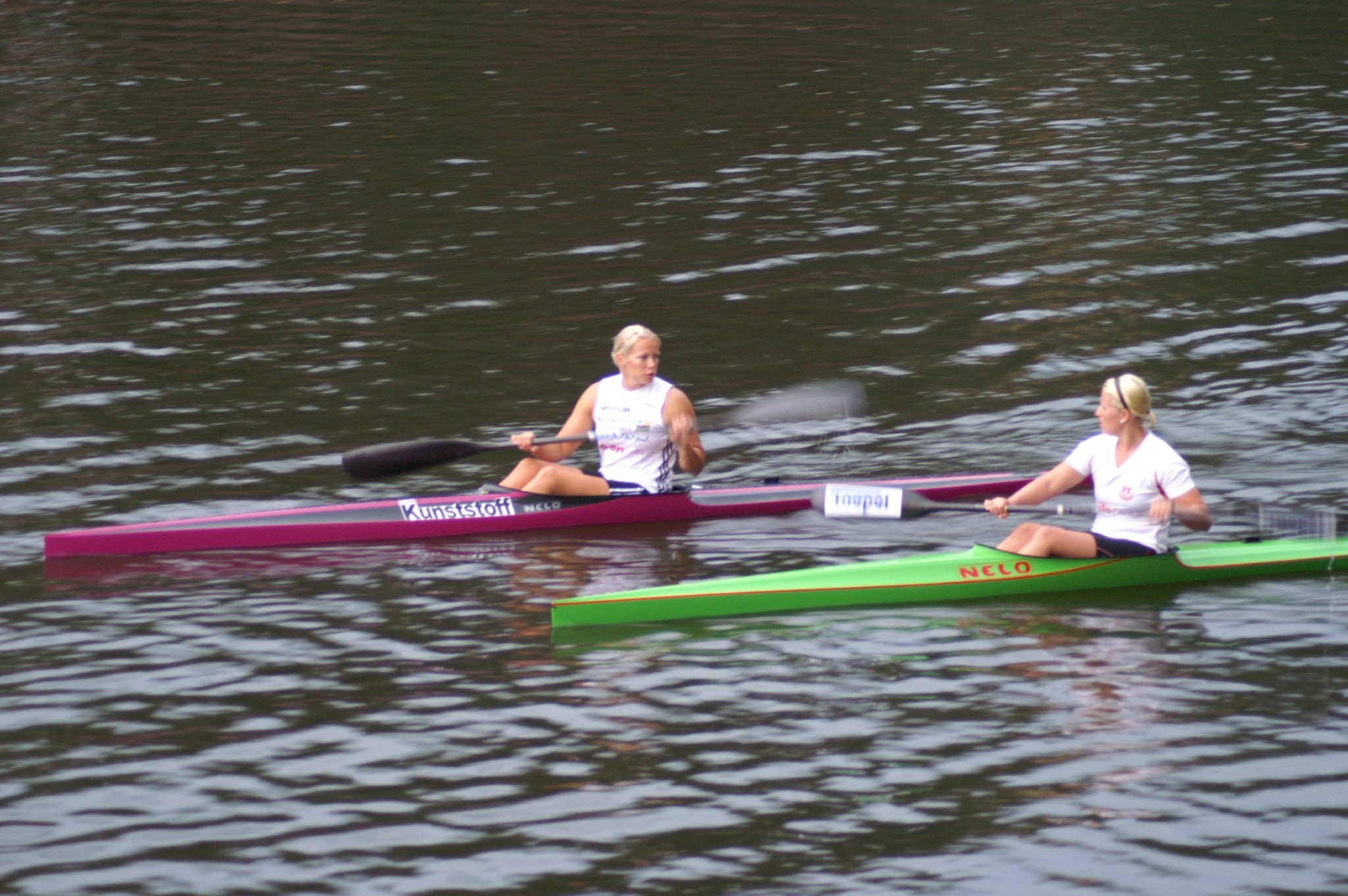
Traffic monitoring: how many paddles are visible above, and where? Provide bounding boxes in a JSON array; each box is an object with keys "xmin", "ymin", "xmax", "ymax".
[
  {"xmin": 342, "ymin": 376, "xmax": 867, "ymax": 482},
  {"xmin": 810, "ymin": 479, "xmax": 1348, "ymax": 549}
]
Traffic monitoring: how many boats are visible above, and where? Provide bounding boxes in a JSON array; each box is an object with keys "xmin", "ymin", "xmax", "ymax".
[
  {"xmin": 44, "ymin": 470, "xmax": 1093, "ymax": 560},
  {"xmin": 552, "ymin": 537, "xmax": 1348, "ymax": 630}
]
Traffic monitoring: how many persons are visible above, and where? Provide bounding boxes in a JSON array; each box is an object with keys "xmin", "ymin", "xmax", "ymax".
[
  {"xmin": 984, "ymin": 374, "xmax": 1214, "ymax": 560},
  {"xmin": 498, "ymin": 325, "xmax": 704, "ymax": 495}
]
